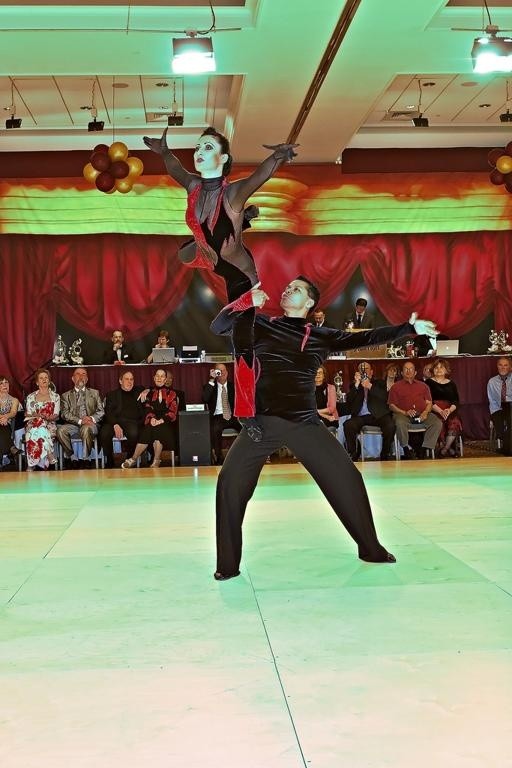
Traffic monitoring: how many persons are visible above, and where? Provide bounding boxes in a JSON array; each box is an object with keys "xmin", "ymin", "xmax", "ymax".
[
  {"xmin": 24, "ymin": 368, "xmax": 60, "ymax": 471},
  {"xmin": 142, "ymin": 331, "xmax": 180, "ymax": 364},
  {"xmin": 204, "ymin": 363, "xmax": 243, "ymax": 465},
  {"xmin": 104, "ymin": 329, "xmax": 139, "ymax": 364},
  {"xmin": 313, "ymin": 360, "xmax": 463, "ymax": 462},
  {"xmin": 415, "ymin": 320, "xmax": 450, "ymax": 357},
  {"xmin": 99, "ymin": 369, "xmax": 185, "ymax": 468},
  {"xmin": 209, "ymin": 275, "xmax": 441, "ymax": 580},
  {"xmin": 1, "ymin": 375, "xmax": 23, "ymax": 472},
  {"xmin": 343, "ymin": 298, "xmax": 371, "ymax": 329},
  {"xmin": 142, "ymin": 126, "xmax": 299, "ymax": 443},
  {"xmin": 56, "ymin": 368, "xmax": 104, "ymax": 463},
  {"xmin": 309, "ymin": 307, "xmax": 335, "ymax": 328},
  {"xmin": 488, "ymin": 358, "xmax": 512, "ymax": 456}
]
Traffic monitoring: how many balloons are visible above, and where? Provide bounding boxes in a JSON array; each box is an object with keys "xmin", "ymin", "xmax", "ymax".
[
  {"xmin": 82, "ymin": 141, "xmax": 143, "ymax": 195},
  {"xmin": 488, "ymin": 141, "xmax": 512, "ymax": 195}
]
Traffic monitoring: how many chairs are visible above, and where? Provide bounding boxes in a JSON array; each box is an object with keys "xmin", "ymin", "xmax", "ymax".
[
  {"xmin": 19, "ymin": 435, "xmax": 58, "ymax": 471},
  {"xmin": 59, "ymin": 437, "xmax": 99, "ymax": 469},
  {"xmin": 397, "ymin": 423, "xmax": 435, "ymax": 460},
  {"xmin": 488, "ymin": 418, "xmax": 505, "ymax": 450},
  {"xmin": 100, "ymin": 436, "xmax": 127, "ymax": 470},
  {"xmin": 138, "ymin": 450, "xmax": 176, "ymax": 468},
  {"xmin": 360, "ymin": 424, "xmax": 397, "ymax": 460},
  {"xmin": 455, "ymin": 429, "xmax": 464, "ymax": 457},
  {"xmin": 211, "ymin": 428, "xmax": 240, "ymax": 464}
]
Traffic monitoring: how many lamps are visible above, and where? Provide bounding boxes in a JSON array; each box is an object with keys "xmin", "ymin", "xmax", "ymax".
[
  {"xmin": 6, "ymin": 78, "xmax": 183, "ymax": 129},
  {"xmin": 172, "ymin": 0, "xmax": 216, "ymax": 74},
  {"xmin": 471, "ymin": 0, "xmax": 512, "ymax": 75},
  {"xmin": 412, "ymin": 78, "xmax": 512, "ymax": 127}
]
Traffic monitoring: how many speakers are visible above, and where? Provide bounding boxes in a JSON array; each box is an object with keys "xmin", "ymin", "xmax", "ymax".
[{"xmin": 178, "ymin": 410, "xmax": 211, "ymax": 466}]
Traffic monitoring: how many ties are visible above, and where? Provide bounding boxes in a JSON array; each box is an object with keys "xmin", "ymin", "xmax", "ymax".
[
  {"xmin": 77, "ymin": 389, "xmax": 87, "ymax": 418},
  {"xmin": 501, "ymin": 378, "xmax": 507, "ymax": 402},
  {"xmin": 221, "ymin": 385, "xmax": 231, "ymax": 421}
]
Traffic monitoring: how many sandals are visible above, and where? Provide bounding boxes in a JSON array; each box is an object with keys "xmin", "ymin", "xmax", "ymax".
[{"xmin": 121, "ymin": 459, "xmax": 136, "ymax": 470}]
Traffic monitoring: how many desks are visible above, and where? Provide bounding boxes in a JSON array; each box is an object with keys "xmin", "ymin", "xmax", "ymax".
[{"xmin": 51, "ymin": 354, "xmax": 512, "ymax": 406}]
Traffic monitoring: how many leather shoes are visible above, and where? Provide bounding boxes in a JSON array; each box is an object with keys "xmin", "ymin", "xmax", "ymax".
[
  {"xmin": 451, "ymin": 452, "xmax": 460, "ymax": 458},
  {"xmin": 50, "ymin": 459, "xmax": 58, "ymax": 464},
  {"xmin": 438, "ymin": 448, "xmax": 445, "ymax": 459}
]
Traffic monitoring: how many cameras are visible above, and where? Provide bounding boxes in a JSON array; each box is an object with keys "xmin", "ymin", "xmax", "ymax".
[
  {"xmin": 215, "ymin": 370, "xmax": 222, "ymax": 376},
  {"xmin": 411, "ymin": 416, "xmax": 421, "ymax": 423},
  {"xmin": 360, "ymin": 362, "xmax": 367, "ymax": 380}
]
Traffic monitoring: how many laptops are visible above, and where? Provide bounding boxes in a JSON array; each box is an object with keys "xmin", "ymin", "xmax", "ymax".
[
  {"xmin": 152, "ymin": 348, "xmax": 174, "ymax": 363},
  {"xmin": 436, "ymin": 340, "xmax": 459, "ymax": 356}
]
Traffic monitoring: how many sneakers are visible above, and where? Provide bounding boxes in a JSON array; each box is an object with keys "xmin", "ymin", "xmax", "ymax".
[
  {"xmin": 71, "ymin": 455, "xmax": 78, "ymax": 462},
  {"xmin": 243, "ymin": 205, "xmax": 259, "ymax": 221},
  {"xmin": 238, "ymin": 418, "xmax": 263, "ymax": 442},
  {"xmin": 83, "ymin": 456, "xmax": 91, "ymax": 462}
]
